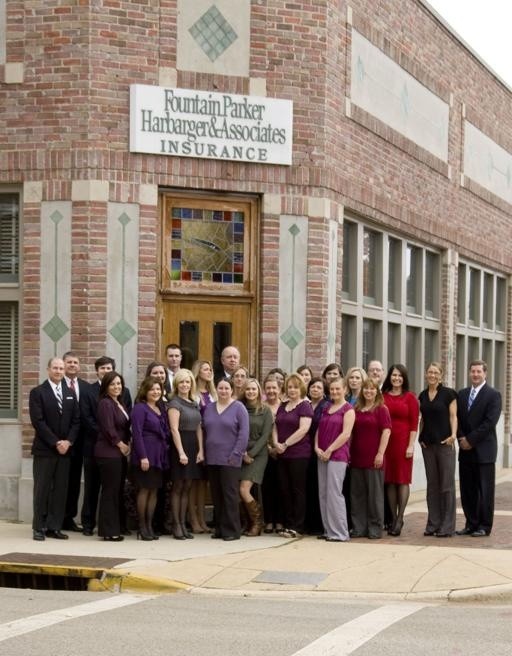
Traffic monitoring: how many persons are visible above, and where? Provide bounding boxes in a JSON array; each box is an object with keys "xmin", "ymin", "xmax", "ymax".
[
  {"xmin": 28, "ymin": 352, "xmax": 419, "ymax": 543},
  {"xmin": 164, "ymin": 344, "xmax": 182, "ymax": 393},
  {"xmin": 213, "ymin": 346, "xmax": 240, "ymax": 390},
  {"xmin": 417, "ymin": 363, "xmax": 460, "ymax": 538},
  {"xmin": 454, "ymin": 359, "xmax": 502, "ymax": 538}
]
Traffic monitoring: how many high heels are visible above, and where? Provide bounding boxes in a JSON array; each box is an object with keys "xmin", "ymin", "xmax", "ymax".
[{"xmin": 137, "ymin": 501, "xmax": 215, "ymax": 540}]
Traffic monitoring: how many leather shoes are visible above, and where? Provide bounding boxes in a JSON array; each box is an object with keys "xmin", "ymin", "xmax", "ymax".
[
  {"xmin": 104, "ymin": 530, "xmax": 132, "ymax": 541},
  {"xmin": 317, "ymin": 519, "xmax": 405, "ymax": 543},
  {"xmin": 423, "ymin": 526, "xmax": 488, "ymax": 537},
  {"xmin": 33, "ymin": 525, "xmax": 94, "ymax": 540},
  {"xmin": 210, "ymin": 500, "xmax": 305, "ymax": 542}
]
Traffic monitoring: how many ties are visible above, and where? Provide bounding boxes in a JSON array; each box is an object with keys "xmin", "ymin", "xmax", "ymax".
[
  {"xmin": 56, "ymin": 384, "xmax": 63, "ymax": 416},
  {"xmin": 466, "ymin": 387, "xmax": 477, "ymax": 409},
  {"xmin": 70, "ymin": 379, "xmax": 77, "ymax": 392}
]
